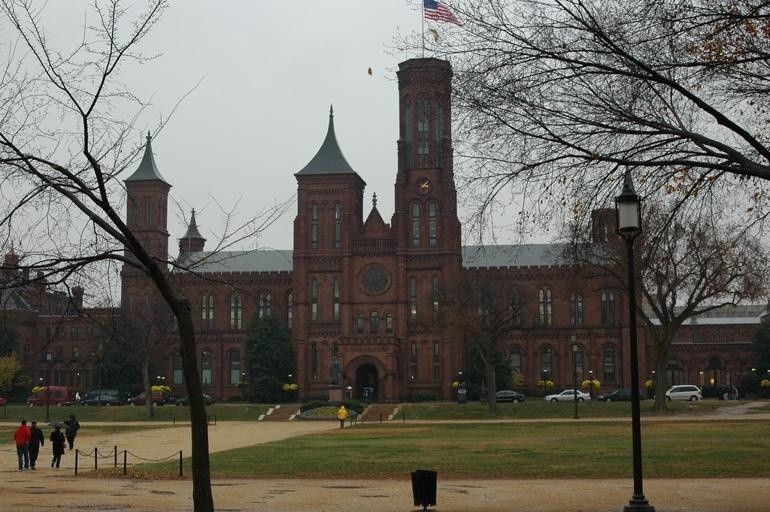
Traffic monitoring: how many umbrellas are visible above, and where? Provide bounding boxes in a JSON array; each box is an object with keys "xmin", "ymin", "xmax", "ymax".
[{"xmin": 48, "ymin": 421, "xmax": 71, "ymax": 430}]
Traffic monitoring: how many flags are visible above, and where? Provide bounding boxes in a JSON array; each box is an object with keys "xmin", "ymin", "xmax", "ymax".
[{"xmin": 424, "ymin": 1, "xmax": 463, "ymax": 27}]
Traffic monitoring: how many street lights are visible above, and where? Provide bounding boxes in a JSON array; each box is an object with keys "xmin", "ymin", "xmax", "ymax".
[
  {"xmin": 46, "ymin": 350, "xmax": 53, "ymax": 422},
  {"xmin": 570, "ymin": 342, "xmax": 578, "ymax": 419},
  {"xmin": 614, "ymin": 173, "xmax": 655, "ymax": 512}
]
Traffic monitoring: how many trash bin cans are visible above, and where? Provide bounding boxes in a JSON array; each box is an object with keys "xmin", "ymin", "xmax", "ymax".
[
  {"xmin": 457, "ymin": 389, "xmax": 467, "ymax": 403},
  {"xmin": 410, "ymin": 468, "xmax": 437, "ymax": 511}
]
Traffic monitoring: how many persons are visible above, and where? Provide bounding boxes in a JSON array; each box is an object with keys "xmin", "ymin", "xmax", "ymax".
[
  {"xmin": 364, "ymin": 389, "xmax": 370, "ymax": 400},
  {"xmin": 651, "ymin": 384, "xmax": 656, "ymax": 398},
  {"xmin": 13, "ymin": 418, "xmax": 32, "ymax": 471},
  {"xmin": 75, "ymin": 392, "xmax": 82, "ymax": 403},
  {"xmin": 50, "ymin": 425, "xmax": 65, "ymax": 467},
  {"xmin": 716, "ymin": 383, "xmax": 741, "ymax": 400},
  {"xmin": 29, "ymin": 420, "xmax": 44, "ymax": 470},
  {"xmin": 337, "ymin": 405, "xmax": 349, "ymax": 428},
  {"xmin": 647, "ymin": 386, "xmax": 652, "ymax": 398},
  {"xmin": 64, "ymin": 415, "xmax": 80, "ymax": 450},
  {"xmin": 698, "ymin": 384, "xmax": 705, "ymax": 400}
]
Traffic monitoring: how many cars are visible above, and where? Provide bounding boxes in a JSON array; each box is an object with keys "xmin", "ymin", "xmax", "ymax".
[
  {"xmin": 81, "ymin": 391, "xmax": 215, "ymax": 406},
  {"xmin": 666, "ymin": 384, "xmax": 702, "ymax": 401},
  {"xmin": 597, "ymin": 388, "xmax": 643, "ymax": 402},
  {"xmin": 496, "ymin": 390, "xmax": 525, "ymax": 404},
  {"xmin": 545, "ymin": 389, "xmax": 591, "ymax": 402}
]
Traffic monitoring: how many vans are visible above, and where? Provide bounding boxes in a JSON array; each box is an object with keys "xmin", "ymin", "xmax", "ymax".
[{"xmin": 26, "ymin": 385, "xmax": 70, "ymax": 408}]
